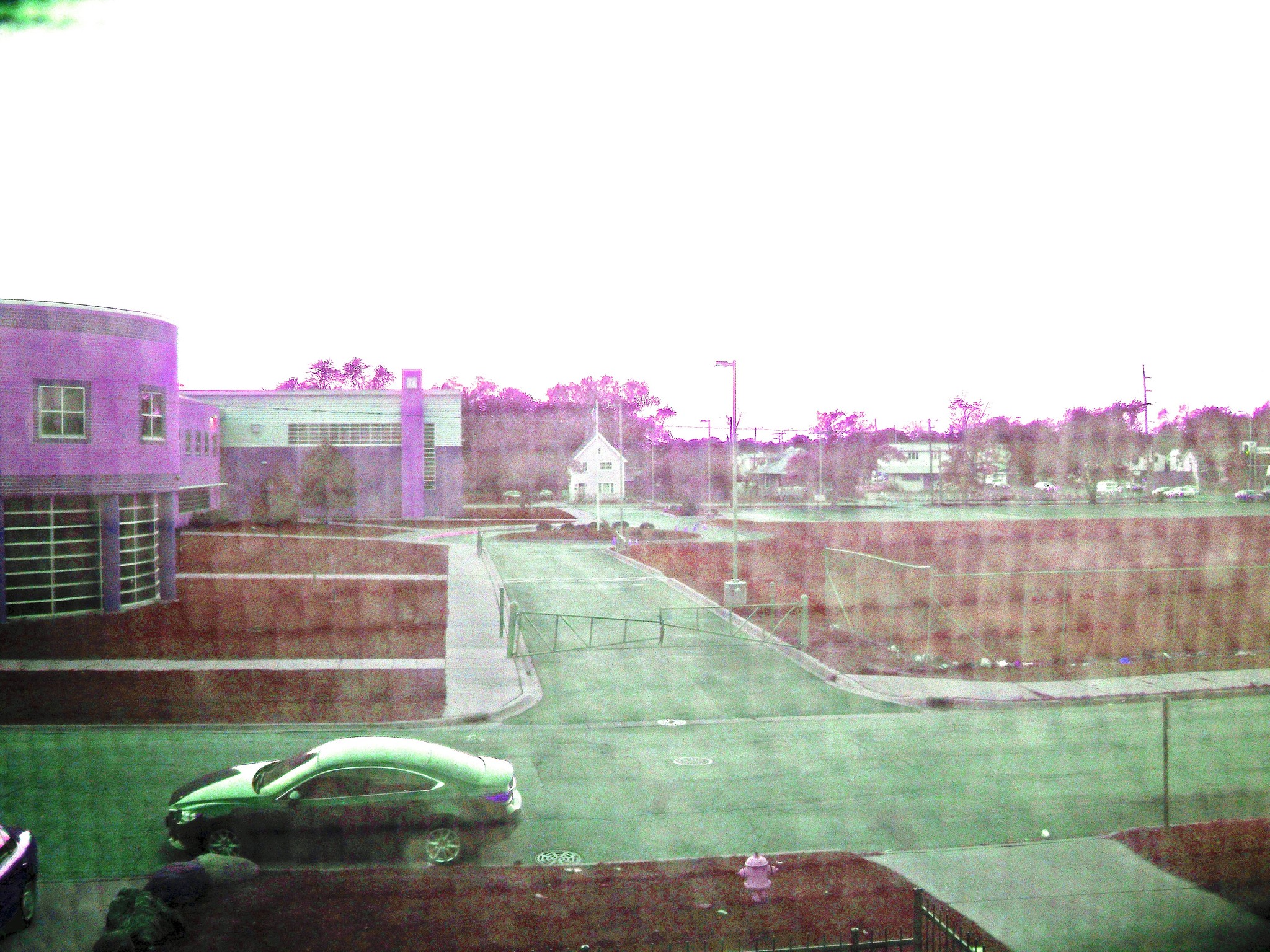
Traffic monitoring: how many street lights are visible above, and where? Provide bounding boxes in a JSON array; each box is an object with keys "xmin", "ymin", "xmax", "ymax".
[{"xmin": 715, "ymin": 359, "xmax": 738, "ymax": 583}]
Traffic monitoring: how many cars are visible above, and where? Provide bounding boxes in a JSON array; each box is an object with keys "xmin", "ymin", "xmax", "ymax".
[
  {"xmin": 1027, "ymin": 479, "xmax": 1263, "ymax": 505},
  {"xmin": 0, "ymin": 823, "xmax": 38, "ymax": 933},
  {"xmin": 165, "ymin": 735, "xmax": 524, "ymax": 867}
]
